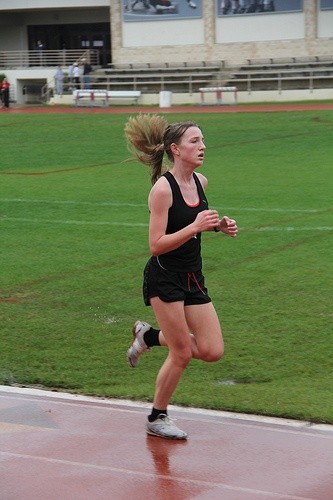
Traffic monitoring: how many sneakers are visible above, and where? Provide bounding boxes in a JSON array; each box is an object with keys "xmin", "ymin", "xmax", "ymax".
[
  {"xmin": 146, "ymin": 413, "xmax": 188, "ymax": 440},
  {"xmin": 128, "ymin": 320, "xmax": 154, "ymax": 368}
]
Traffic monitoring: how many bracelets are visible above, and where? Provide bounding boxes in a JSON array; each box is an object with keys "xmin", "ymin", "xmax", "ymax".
[{"xmin": 214, "ymin": 227, "xmax": 220, "ymax": 232}]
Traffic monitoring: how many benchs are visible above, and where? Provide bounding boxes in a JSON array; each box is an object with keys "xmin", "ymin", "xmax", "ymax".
[{"xmin": 55, "ymin": 61, "xmax": 333, "ymax": 106}]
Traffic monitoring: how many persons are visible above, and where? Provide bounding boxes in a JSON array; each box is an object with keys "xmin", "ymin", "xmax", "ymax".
[
  {"xmin": 127, "ymin": 0, "xmax": 197, "ymax": 13},
  {"xmin": 123, "ymin": 112, "xmax": 238, "ymax": 439},
  {"xmin": 82, "ymin": 59, "xmax": 92, "ymax": 89},
  {"xmin": 73, "ymin": 63, "xmax": 80, "ymax": 89},
  {"xmin": 220, "ymin": 0, "xmax": 275, "ymax": 15},
  {"xmin": 37, "ymin": 41, "xmax": 45, "ymax": 66},
  {"xmin": 0, "ymin": 78, "xmax": 9, "ymax": 108},
  {"xmin": 54, "ymin": 68, "xmax": 64, "ymax": 94}
]
